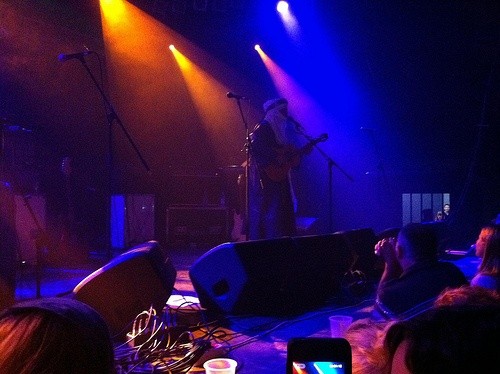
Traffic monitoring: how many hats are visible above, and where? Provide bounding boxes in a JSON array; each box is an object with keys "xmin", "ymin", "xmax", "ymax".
[{"xmin": 262, "ymin": 98, "xmax": 287, "ymax": 112}]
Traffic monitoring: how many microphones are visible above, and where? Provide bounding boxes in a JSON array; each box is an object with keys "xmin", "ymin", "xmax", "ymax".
[
  {"xmin": 7, "ymin": 126, "xmax": 31, "ymax": 132},
  {"xmin": 57, "ymin": 50, "xmax": 94, "ymax": 62},
  {"xmin": 287, "ymin": 116, "xmax": 305, "ymax": 130},
  {"xmin": 227, "ymin": 92, "xmax": 247, "ymax": 100}
]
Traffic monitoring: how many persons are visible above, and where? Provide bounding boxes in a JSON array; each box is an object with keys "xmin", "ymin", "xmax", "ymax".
[
  {"xmin": 375, "ymin": 287, "xmax": 500, "ymax": 374},
  {"xmin": 375, "ymin": 224, "xmax": 472, "ymax": 320},
  {"xmin": 471, "ymin": 225, "xmax": 500, "ymax": 294},
  {"xmin": 249, "ymin": 98, "xmax": 313, "ymax": 239},
  {"xmin": 0, "ymin": 298, "xmax": 115, "ymax": 374},
  {"xmin": 436, "ymin": 204, "xmax": 450, "ymax": 220}
]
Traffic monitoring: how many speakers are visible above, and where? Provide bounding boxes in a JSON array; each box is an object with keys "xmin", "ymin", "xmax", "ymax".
[
  {"xmin": 14, "ymin": 194, "xmax": 47, "ymax": 260},
  {"xmin": 166, "ymin": 205, "xmax": 230, "ymax": 252},
  {"xmin": 168, "ymin": 169, "xmax": 232, "ymax": 208},
  {"xmin": 68, "ymin": 240, "xmax": 177, "ymax": 340},
  {"xmin": 110, "ymin": 193, "xmax": 156, "ymax": 250},
  {"xmin": 189, "ymin": 226, "xmax": 401, "ymax": 319}
]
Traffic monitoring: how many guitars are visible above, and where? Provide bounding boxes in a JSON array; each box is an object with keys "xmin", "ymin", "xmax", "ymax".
[{"xmin": 262, "ymin": 132, "xmax": 329, "ymax": 183}]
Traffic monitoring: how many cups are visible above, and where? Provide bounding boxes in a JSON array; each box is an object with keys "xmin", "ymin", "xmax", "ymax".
[
  {"xmin": 330, "ymin": 314, "xmax": 353, "ymax": 339},
  {"xmin": 202, "ymin": 358, "xmax": 237, "ymax": 374}
]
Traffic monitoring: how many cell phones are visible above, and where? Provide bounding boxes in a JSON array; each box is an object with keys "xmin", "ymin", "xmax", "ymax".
[{"xmin": 286, "ymin": 337, "xmax": 352, "ymax": 374}]
